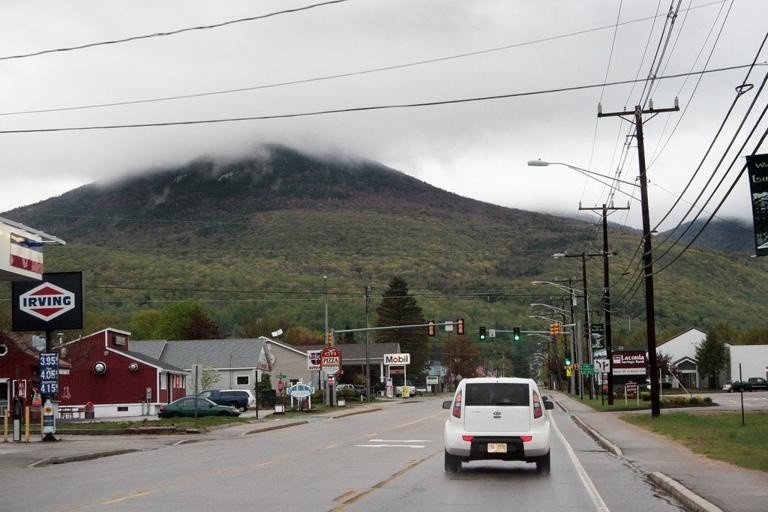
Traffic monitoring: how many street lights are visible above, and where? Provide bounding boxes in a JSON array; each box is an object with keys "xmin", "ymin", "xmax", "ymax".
[
  {"xmin": 323, "ymin": 275, "xmax": 327, "ymax": 346},
  {"xmin": 528, "ymin": 160, "xmax": 659, "ymax": 417},
  {"xmin": 528, "ymin": 280, "xmax": 590, "ymax": 396},
  {"xmin": 553, "ymin": 253, "xmax": 593, "ymax": 399}
]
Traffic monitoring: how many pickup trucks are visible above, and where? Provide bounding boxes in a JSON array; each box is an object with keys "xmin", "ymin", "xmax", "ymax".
[
  {"xmin": 198, "ymin": 390, "xmax": 247, "ymax": 412},
  {"xmin": 733, "ymin": 378, "xmax": 767, "ymax": 392},
  {"xmin": 395, "ymin": 380, "xmax": 416, "ymax": 397},
  {"xmin": 355, "ymin": 385, "xmax": 367, "ymax": 397}
]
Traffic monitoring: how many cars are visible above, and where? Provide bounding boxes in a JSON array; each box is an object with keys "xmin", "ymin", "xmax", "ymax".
[
  {"xmin": 336, "ymin": 384, "xmax": 355, "ymax": 393},
  {"xmin": 722, "ymin": 377, "xmax": 745, "ymax": 393},
  {"xmin": 441, "ymin": 378, "xmax": 553, "ymax": 475},
  {"xmin": 286, "ymin": 384, "xmax": 315, "ymax": 398},
  {"xmin": 206, "ymin": 390, "xmax": 256, "ymax": 412},
  {"xmin": 158, "ymin": 396, "xmax": 240, "ymax": 419}
]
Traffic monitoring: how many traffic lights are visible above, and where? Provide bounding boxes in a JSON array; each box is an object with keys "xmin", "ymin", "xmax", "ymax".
[
  {"xmin": 564, "ymin": 352, "xmax": 571, "ymax": 367},
  {"xmin": 549, "ymin": 322, "xmax": 560, "ymax": 336},
  {"xmin": 514, "ymin": 327, "xmax": 520, "ymax": 342},
  {"xmin": 479, "ymin": 326, "xmax": 486, "ymax": 341}
]
